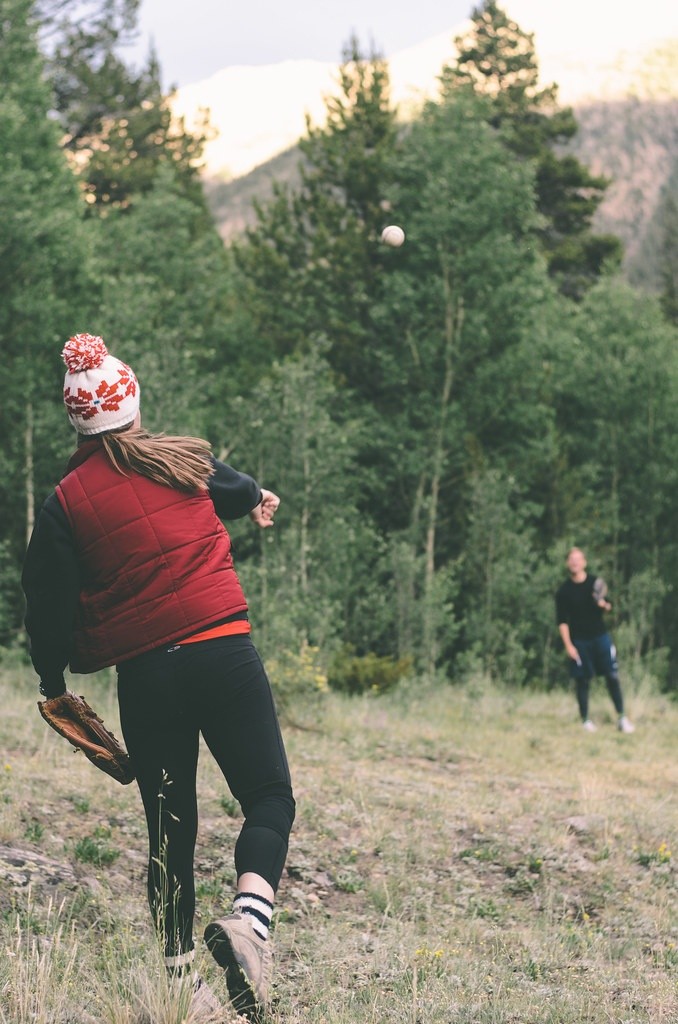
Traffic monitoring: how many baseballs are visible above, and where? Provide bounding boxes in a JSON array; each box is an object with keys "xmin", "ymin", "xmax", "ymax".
[{"xmin": 382, "ymin": 225, "xmax": 405, "ymax": 249}]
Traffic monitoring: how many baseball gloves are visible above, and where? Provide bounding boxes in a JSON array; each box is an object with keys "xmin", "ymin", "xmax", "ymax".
[
  {"xmin": 34, "ymin": 692, "xmax": 136, "ymax": 786},
  {"xmin": 591, "ymin": 577, "xmax": 608, "ymax": 601}
]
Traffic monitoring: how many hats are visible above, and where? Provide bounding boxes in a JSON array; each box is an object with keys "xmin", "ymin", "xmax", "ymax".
[{"xmin": 61, "ymin": 332, "xmax": 141, "ymax": 435}]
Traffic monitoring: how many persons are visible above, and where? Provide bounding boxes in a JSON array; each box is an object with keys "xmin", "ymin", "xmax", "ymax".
[
  {"xmin": 22, "ymin": 333, "xmax": 296, "ymax": 1024},
  {"xmin": 555, "ymin": 546, "xmax": 635, "ymax": 735}
]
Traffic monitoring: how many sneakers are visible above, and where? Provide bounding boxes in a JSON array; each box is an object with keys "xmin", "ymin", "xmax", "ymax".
[
  {"xmin": 203, "ymin": 904, "xmax": 273, "ymax": 1020},
  {"xmin": 194, "ymin": 983, "xmax": 222, "ymax": 1018}
]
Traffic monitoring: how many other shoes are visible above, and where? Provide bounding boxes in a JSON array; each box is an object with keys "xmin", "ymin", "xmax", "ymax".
[
  {"xmin": 618, "ymin": 717, "xmax": 633, "ymax": 733},
  {"xmin": 585, "ymin": 722, "xmax": 597, "ymax": 732}
]
